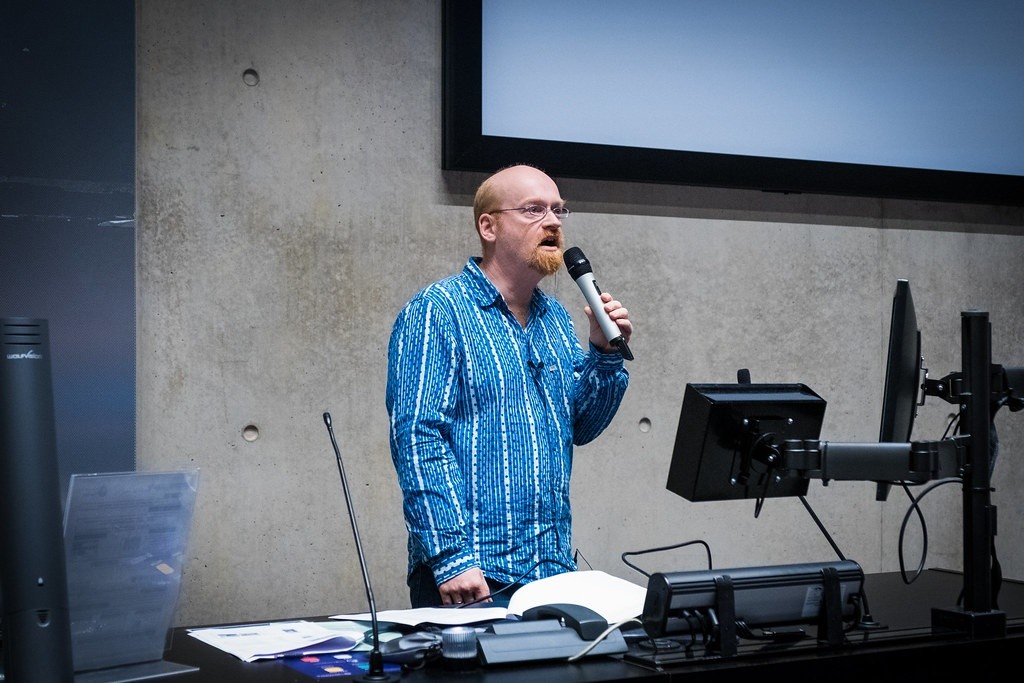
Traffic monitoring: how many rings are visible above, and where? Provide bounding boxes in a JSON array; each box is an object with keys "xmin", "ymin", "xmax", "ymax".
[{"xmin": 454, "ymin": 600, "xmax": 462, "ymax": 604}]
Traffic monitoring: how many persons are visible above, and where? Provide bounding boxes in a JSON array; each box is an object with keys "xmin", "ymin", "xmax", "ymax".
[{"xmin": 385, "ymin": 163, "xmax": 634, "ymax": 609}]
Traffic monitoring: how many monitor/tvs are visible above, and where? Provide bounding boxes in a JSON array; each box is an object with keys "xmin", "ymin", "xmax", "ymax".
[{"xmin": 875, "ymin": 279, "xmax": 921, "ymax": 502}]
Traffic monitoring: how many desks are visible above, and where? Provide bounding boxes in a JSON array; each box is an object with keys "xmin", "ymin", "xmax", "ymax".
[{"xmin": 163, "ymin": 569, "xmax": 1024, "ymax": 683}]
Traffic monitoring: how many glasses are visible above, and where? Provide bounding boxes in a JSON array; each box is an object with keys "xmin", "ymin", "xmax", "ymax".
[{"xmin": 488, "ymin": 205, "xmax": 569, "ymax": 220}]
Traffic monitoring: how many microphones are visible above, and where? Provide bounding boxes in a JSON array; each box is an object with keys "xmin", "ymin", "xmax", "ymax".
[
  {"xmin": 563, "ymin": 247, "xmax": 635, "ymax": 361},
  {"xmin": 323, "ymin": 413, "xmax": 388, "ymax": 683}
]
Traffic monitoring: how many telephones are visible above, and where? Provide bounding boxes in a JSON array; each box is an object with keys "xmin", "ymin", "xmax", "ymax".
[{"xmin": 476, "ymin": 603, "xmax": 630, "ymax": 663}]
